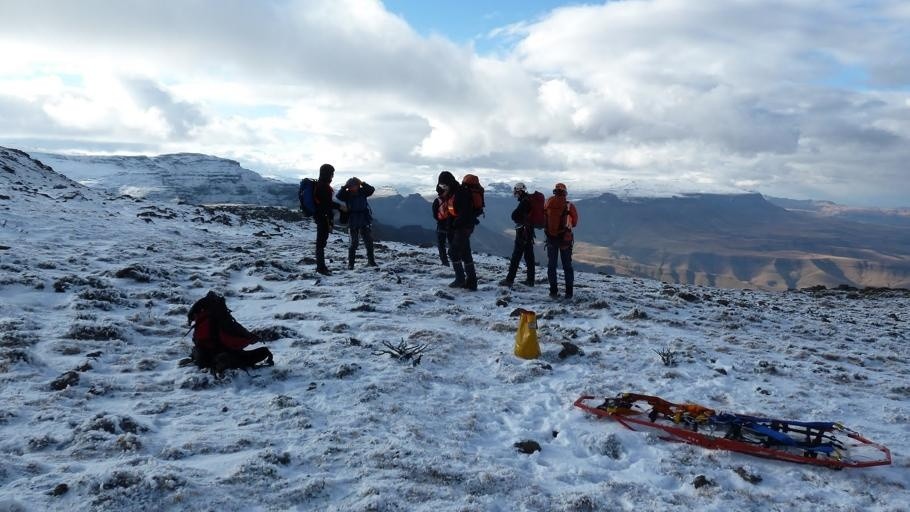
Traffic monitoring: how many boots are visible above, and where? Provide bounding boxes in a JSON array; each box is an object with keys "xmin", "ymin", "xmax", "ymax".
[
  {"xmin": 316, "ymin": 260, "xmax": 333, "ymax": 277},
  {"xmin": 347, "ymin": 247, "xmax": 380, "ymax": 271}
]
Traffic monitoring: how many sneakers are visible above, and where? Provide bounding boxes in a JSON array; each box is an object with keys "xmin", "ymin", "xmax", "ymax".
[
  {"xmin": 498, "ymin": 278, "xmax": 536, "ymax": 289},
  {"xmin": 550, "ymin": 285, "xmax": 574, "ymax": 299},
  {"xmin": 448, "ymin": 276, "xmax": 478, "ymax": 290}
]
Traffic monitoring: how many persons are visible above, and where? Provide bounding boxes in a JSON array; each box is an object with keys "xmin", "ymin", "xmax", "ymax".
[
  {"xmin": 438, "ymin": 170, "xmax": 478, "ymax": 292},
  {"xmin": 432, "ymin": 184, "xmax": 452, "ymax": 268},
  {"xmin": 497, "ymin": 182, "xmax": 535, "ymax": 289},
  {"xmin": 336, "ymin": 176, "xmax": 379, "ymax": 271},
  {"xmin": 543, "ymin": 182, "xmax": 578, "ymax": 298},
  {"xmin": 312, "ymin": 163, "xmax": 347, "ymax": 277}
]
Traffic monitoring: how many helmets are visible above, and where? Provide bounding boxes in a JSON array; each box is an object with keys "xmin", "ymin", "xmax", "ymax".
[
  {"xmin": 513, "ymin": 182, "xmax": 526, "ymax": 195},
  {"xmin": 551, "ymin": 182, "xmax": 568, "ymax": 195}
]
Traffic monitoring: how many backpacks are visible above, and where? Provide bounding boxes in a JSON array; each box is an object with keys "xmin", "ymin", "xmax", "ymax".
[
  {"xmin": 461, "ymin": 174, "xmax": 486, "ymax": 218},
  {"xmin": 297, "ymin": 176, "xmax": 318, "ymax": 218},
  {"xmin": 544, "ymin": 194, "xmax": 572, "ymax": 243},
  {"xmin": 528, "ymin": 190, "xmax": 546, "ymax": 231}
]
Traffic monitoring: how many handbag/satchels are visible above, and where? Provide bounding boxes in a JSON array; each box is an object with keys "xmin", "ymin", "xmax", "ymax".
[{"xmin": 513, "ymin": 312, "xmax": 544, "ymax": 359}]
